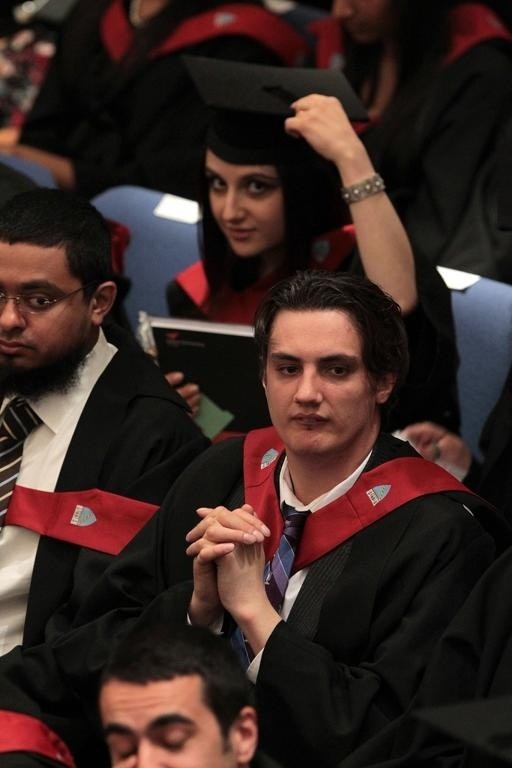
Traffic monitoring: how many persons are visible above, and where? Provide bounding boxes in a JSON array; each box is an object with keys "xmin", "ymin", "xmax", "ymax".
[
  {"xmin": 0, "ymin": 180, "xmax": 216, "ymax": 650},
  {"xmin": 400, "ymin": 365, "xmax": 511, "ymax": 526},
  {"xmin": 160, "ymin": 89, "xmax": 464, "ymax": 429},
  {"xmin": 0, "ymin": 0, "xmax": 512, "ymax": 283},
  {"xmin": 0, "ymin": 264, "xmax": 508, "ymax": 767},
  {"xmin": 96, "ymin": 612, "xmax": 263, "ymax": 767}
]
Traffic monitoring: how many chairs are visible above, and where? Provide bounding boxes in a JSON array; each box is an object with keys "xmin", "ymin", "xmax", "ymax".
[
  {"xmin": 282, "ymin": 6, "xmax": 331, "ymax": 48},
  {"xmin": 435, "ymin": 263, "xmax": 511, "ymax": 485},
  {"xmin": 0, "ymin": 151, "xmax": 60, "ymax": 192},
  {"xmin": 90, "ymin": 184, "xmax": 204, "ymax": 329}
]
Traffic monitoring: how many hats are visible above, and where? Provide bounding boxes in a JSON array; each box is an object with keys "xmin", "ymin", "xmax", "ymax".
[{"xmin": 173, "ymin": 54, "xmax": 370, "ymax": 165}]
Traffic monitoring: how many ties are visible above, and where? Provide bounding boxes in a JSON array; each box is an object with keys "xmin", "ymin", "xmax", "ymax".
[
  {"xmin": 0, "ymin": 396, "xmax": 44, "ymax": 536},
  {"xmin": 229, "ymin": 501, "xmax": 312, "ymax": 672}
]
